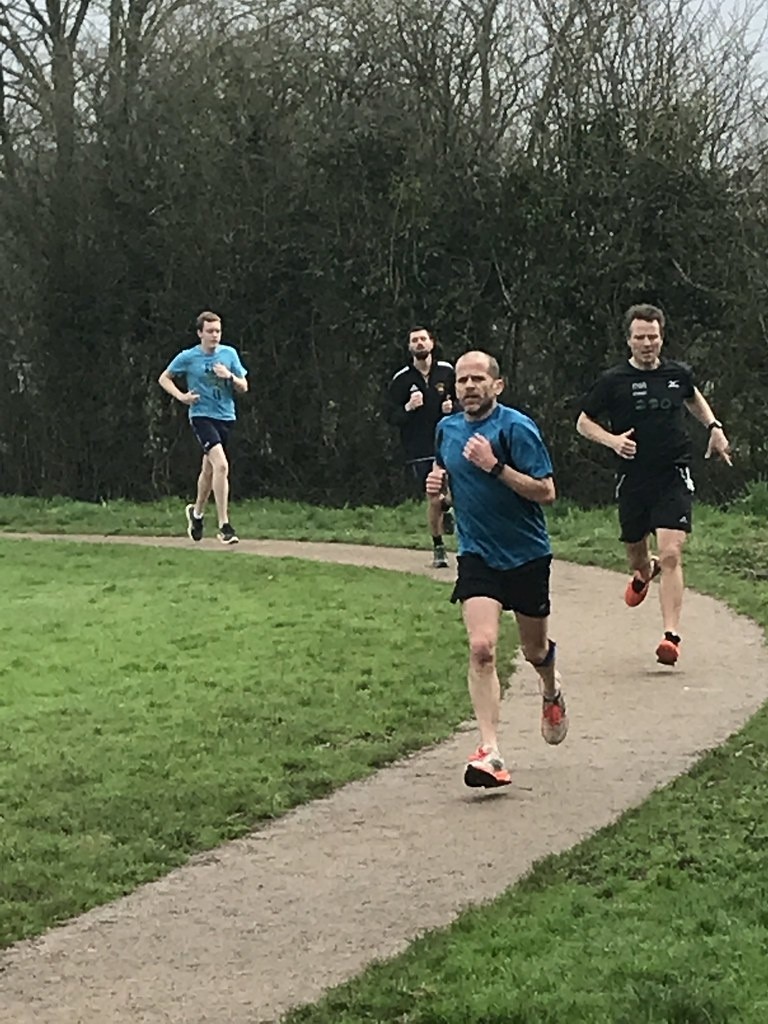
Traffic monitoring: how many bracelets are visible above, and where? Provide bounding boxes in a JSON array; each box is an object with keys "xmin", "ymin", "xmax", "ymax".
[{"xmin": 229, "ymin": 374, "xmax": 233, "ymax": 381}]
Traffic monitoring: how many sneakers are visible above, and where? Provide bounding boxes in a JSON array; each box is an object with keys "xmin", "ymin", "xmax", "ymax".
[
  {"xmin": 539, "ymin": 669, "xmax": 569, "ymax": 746},
  {"xmin": 443, "ymin": 507, "xmax": 457, "ymax": 535},
  {"xmin": 656, "ymin": 632, "xmax": 681, "ymax": 666},
  {"xmin": 185, "ymin": 504, "xmax": 204, "ymax": 541},
  {"xmin": 216, "ymin": 523, "xmax": 239, "ymax": 545},
  {"xmin": 433, "ymin": 544, "xmax": 449, "ymax": 568},
  {"xmin": 625, "ymin": 556, "xmax": 661, "ymax": 607},
  {"xmin": 464, "ymin": 745, "xmax": 512, "ymax": 788}
]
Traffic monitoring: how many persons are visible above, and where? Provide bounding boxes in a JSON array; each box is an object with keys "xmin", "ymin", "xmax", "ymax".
[
  {"xmin": 385, "ymin": 326, "xmax": 464, "ymax": 536},
  {"xmin": 158, "ymin": 312, "xmax": 248, "ymax": 545},
  {"xmin": 426, "ymin": 350, "xmax": 569, "ymax": 790},
  {"xmin": 576, "ymin": 304, "xmax": 733, "ymax": 666}
]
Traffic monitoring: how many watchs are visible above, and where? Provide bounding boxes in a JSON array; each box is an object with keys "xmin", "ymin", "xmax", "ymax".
[
  {"xmin": 708, "ymin": 420, "xmax": 723, "ymax": 430},
  {"xmin": 490, "ymin": 460, "xmax": 504, "ymax": 478}
]
